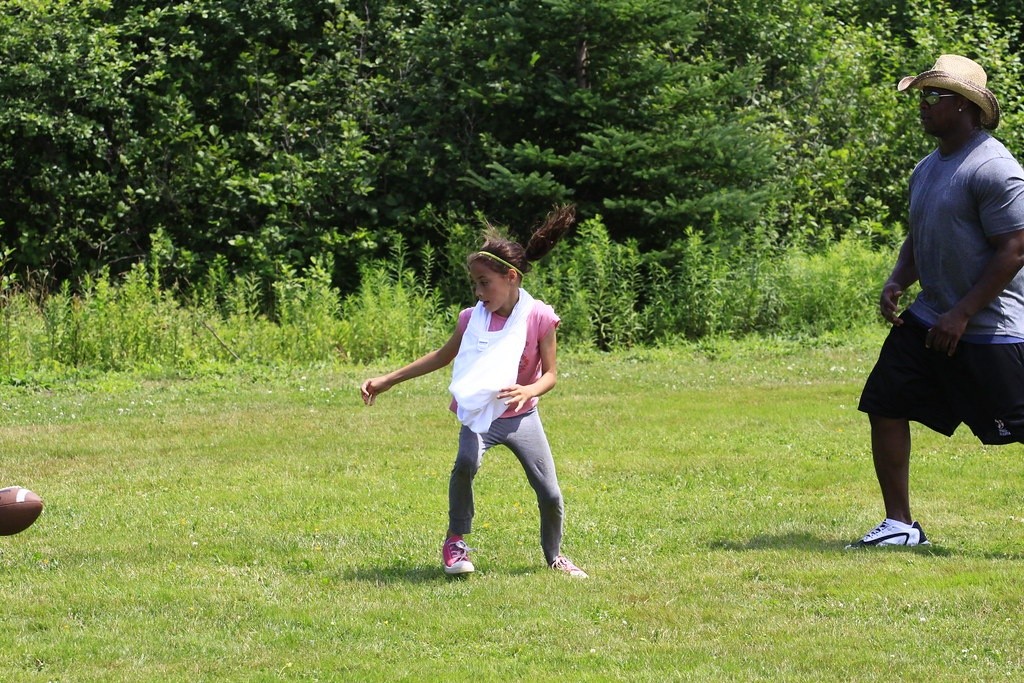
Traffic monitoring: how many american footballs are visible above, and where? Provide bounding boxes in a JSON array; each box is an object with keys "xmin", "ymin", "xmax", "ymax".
[{"xmin": 0, "ymin": 484, "xmax": 43, "ymax": 535}]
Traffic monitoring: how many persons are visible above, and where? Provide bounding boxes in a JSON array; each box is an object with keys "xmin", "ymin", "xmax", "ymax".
[
  {"xmin": 359, "ymin": 205, "xmax": 591, "ymax": 583},
  {"xmin": 846, "ymin": 54, "xmax": 1023, "ymax": 552}
]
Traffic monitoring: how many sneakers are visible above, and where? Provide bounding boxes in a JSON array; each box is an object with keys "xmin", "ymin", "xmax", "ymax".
[
  {"xmin": 550, "ymin": 556, "xmax": 589, "ymax": 579},
  {"xmin": 441, "ymin": 536, "xmax": 474, "ymax": 574},
  {"xmin": 844, "ymin": 518, "xmax": 930, "ymax": 549}
]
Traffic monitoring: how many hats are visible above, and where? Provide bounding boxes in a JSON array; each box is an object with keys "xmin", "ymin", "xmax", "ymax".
[{"xmin": 897, "ymin": 54, "xmax": 1001, "ymax": 130}]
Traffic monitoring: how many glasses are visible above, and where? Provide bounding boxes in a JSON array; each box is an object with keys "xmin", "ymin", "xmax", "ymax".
[{"xmin": 919, "ymin": 89, "xmax": 958, "ymax": 106}]
